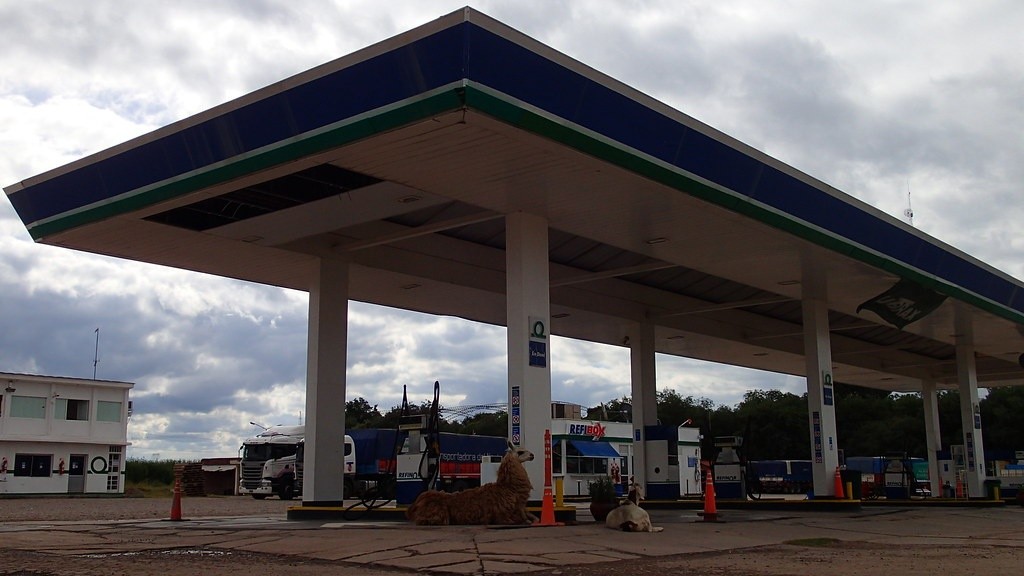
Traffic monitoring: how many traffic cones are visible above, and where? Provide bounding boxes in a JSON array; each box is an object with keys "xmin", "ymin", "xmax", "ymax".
[
  {"xmin": 704, "ymin": 469, "xmax": 717, "ymax": 526},
  {"xmin": 832, "ymin": 467, "xmax": 845, "ymax": 499}
]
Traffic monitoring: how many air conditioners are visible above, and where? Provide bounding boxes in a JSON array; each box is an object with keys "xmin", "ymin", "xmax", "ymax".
[{"xmin": 128, "ymin": 400, "xmax": 133, "ymax": 416}]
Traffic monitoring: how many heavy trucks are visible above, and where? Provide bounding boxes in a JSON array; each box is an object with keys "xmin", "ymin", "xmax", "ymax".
[{"xmin": 238, "ymin": 427, "xmax": 509, "ymax": 501}]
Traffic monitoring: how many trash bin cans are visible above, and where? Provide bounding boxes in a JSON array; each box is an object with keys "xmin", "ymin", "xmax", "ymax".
[{"xmin": 614, "ymin": 484, "xmax": 623, "ymax": 496}]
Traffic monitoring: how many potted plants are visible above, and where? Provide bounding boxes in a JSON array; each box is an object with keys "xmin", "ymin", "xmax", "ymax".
[{"xmin": 587, "ymin": 475, "xmax": 620, "ymax": 520}]
